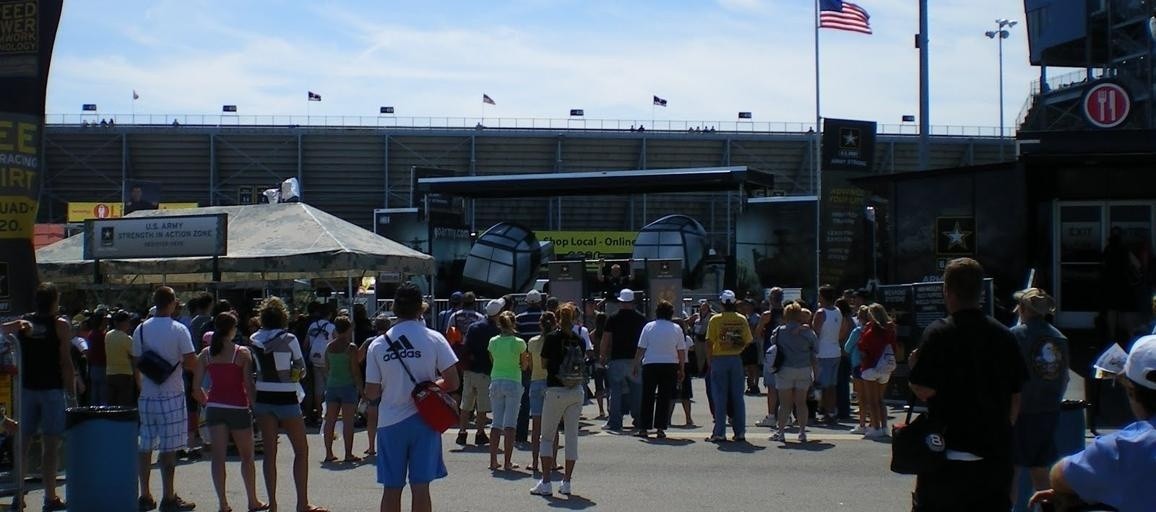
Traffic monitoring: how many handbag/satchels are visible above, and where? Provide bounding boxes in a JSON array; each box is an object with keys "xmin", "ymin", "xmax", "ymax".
[
  {"xmin": 411, "ymin": 381, "xmax": 459, "ymax": 433},
  {"xmin": 136, "ymin": 350, "xmax": 175, "ymax": 384},
  {"xmin": 764, "ymin": 343, "xmax": 782, "ymax": 375},
  {"xmin": 890, "ymin": 413, "xmax": 985, "ymax": 475}
]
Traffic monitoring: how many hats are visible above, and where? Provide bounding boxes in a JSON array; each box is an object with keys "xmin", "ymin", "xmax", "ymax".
[
  {"xmin": 450, "ymin": 291, "xmax": 462, "ymax": 303},
  {"xmin": 617, "ymin": 289, "xmax": 634, "ymax": 302},
  {"xmin": 720, "ymin": 290, "xmax": 736, "ymax": 304},
  {"xmin": 1124, "ymin": 334, "xmax": 1156, "ymax": 389},
  {"xmin": 1013, "ymin": 287, "xmax": 1057, "ymax": 315},
  {"xmin": 524, "ymin": 289, "xmax": 541, "ymax": 303},
  {"xmin": 463, "ymin": 292, "xmax": 475, "ymax": 303},
  {"xmin": 485, "ymin": 297, "xmax": 506, "ymax": 316}
]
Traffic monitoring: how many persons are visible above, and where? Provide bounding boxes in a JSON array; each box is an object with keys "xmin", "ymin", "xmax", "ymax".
[
  {"xmin": 131, "ymin": 287, "xmax": 196, "ymax": 512},
  {"xmin": 908, "ymin": 257, "xmax": 1030, "ymax": 512},
  {"xmin": 1027, "ymin": 334, "xmax": 1156, "ymax": 512},
  {"xmin": 248, "ymin": 295, "xmax": 331, "ymax": 512},
  {"xmin": 172, "ymin": 118, "xmax": 179, "ymax": 127},
  {"xmin": 1007, "ymin": 288, "xmax": 1070, "ymax": 511},
  {"xmin": 365, "ymin": 280, "xmax": 461, "ymax": 511},
  {"xmin": 11, "ymin": 282, "xmax": 76, "ymax": 511},
  {"xmin": 123, "ymin": 185, "xmax": 158, "ymax": 216},
  {"xmin": 83, "ymin": 118, "xmax": 114, "ymax": 128},
  {"xmin": 3, "ymin": 286, "xmax": 897, "ymax": 469},
  {"xmin": 530, "ymin": 305, "xmax": 586, "ymax": 495},
  {"xmin": 192, "ymin": 312, "xmax": 270, "ymax": 512}
]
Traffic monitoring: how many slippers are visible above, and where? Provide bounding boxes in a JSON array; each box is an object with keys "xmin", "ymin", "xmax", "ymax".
[{"xmin": 218, "ymin": 448, "xmax": 376, "ymax": 512}]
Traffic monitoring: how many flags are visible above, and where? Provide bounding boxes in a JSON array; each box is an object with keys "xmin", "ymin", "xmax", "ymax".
[
  {"xmin": 483, "ymin": 94, "xmax": 496, "ymax": 105},
  {"xmin": 654, "ymin": 95, "xmax": 667, "ymax": 108},
  {"xmin": 308, "ymin": 90, "xmax": 321, "ymax": 101},
  {"xmin": 133, "ymin": 89, "xmax": 139, "ymax": 100},
  {"xmin": 820, "ymin": 0, "xmax": 873, "ymax": 34}
]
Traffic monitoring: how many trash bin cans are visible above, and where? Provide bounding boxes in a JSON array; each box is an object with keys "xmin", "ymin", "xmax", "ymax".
[
  {"xmin": 64, "ymin": 405, "xmax": 138, "ymax": 512},
  {"xmin": 1056, "ymin": 399, "xmax": 1092, "ymax": 455}
]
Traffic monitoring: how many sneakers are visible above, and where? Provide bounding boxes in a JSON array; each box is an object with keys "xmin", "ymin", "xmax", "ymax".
[
  {"xmin": 139, "ymin": 493, "xmax": 156, "ymax": 512},
  {"xmin": 594, "ymin": 385, "xmax": 889, "ymax": 442},
  {"xmin": 42, "ymin": 495, "xmax": 67, "ymax": 512},
  {"xmin": 158, "ymin": 492, "xmax": 196, "ymax": 512},
  {"xmin": 456, "ymin": 430, "xmax": 571, "ymax": 495}
]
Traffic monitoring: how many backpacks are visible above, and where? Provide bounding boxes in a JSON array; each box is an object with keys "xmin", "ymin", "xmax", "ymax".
[
  {"xmin": 555, "ymin": 346, "xmax": 588, "ymax": 387},
  {"xmin": 876, "ymin": 345, "xmax": 896, "ymax": 374},
  {"xmin": 310, "ymin": 321, "xmax": 331, "ymax": 368}
]
